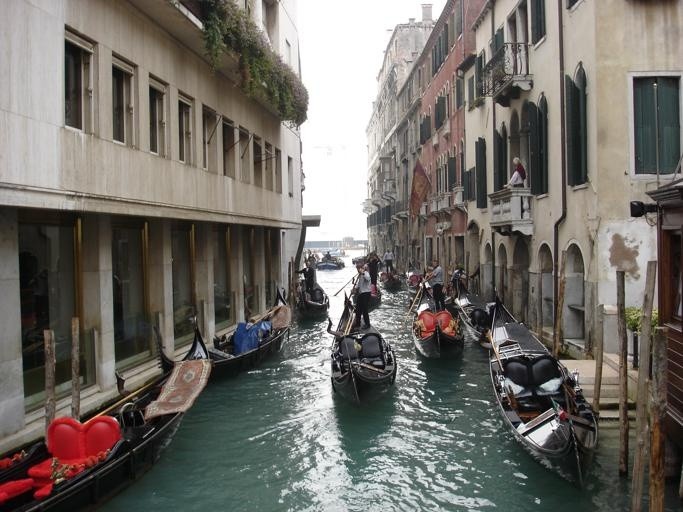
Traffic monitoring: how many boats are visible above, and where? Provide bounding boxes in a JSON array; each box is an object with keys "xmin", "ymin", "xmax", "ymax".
[
  {"xmin": 316, "ymin": 256, "xmax": 345, "ymax": 270},
  {"xmin": 411, "ymin": 301, "xmax": 465, "ymax": 361},
  {"xmin": 301, "ymin": 279, "xmax": 330, "ymax": 313},
  {"xmin": 113, "ymin": 285, "xmax": 292, "ymax": 399},
  {"xmin": 379, "ymin": 271, "xmax": 403, "ymax": 290},
  {"xmin": 342, "ymin": 304, "xmax": 398, "ymax": 386},
  {"xmin": 454, "ymin": 294, "xmax": 491, "ymax": 349},
  {"xmin": 488, "ymin": 286, "xmax": 599, "ymax": 492},
  {"xmin": 0, "ymin": 315, "xmax": 214, "ymax": 512}
]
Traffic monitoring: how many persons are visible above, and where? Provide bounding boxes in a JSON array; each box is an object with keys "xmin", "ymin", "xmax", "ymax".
[
  {"xmin": 350, "ymin": 263, "xmax": 372, "ymax": 330},
  {"xmin": 469, "ymin": 266, "xmax": 480, "ymax": 281},
  {"xmin": 325, "ymin": 251, "xmax": 332, "ymax": 259},
  {"xmin": 382, "ymin": 248, "xmax": 394, "ymax": 274},
  {"xmin": 425, "ymin": 260, "xmax": 445, "ymax": 313},
  {"xmin": 295, "ymin": 261, "xmax": 315, "ymax": 295},
  {"xmin": 366, "ymin": 253, "xmax": 381, "ymax": 293},
  {"xmin": 503, "ymin": 157, "xmax": 527, "ymax": 219},
  {"xmin": 448, "ymin": 267, "xmax": 465, "ymax": 304}
]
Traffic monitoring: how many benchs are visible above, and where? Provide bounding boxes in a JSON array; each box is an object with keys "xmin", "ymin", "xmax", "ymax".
[
  {"xmin": 26, "ymin": 416, "xmax": 119, "ymax": 484},
  {"xmin": 419, "ymin": 311, "xmax": 454, "ymax": 337},
  {"xmin": 502, "ymin": 355, "xmax": 563, "ymax": 406},
  {"xmin": 338, "ymin": 333, "xmax": 385, "ymax": 371},
  {"xmin": 302, "ymin": 289, "xmax": 324, "ymax": 302}
]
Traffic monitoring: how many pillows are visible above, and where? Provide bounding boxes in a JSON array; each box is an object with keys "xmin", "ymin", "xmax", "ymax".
[
  {"xmin": 415, "ymin": 320, "xmax": 426, "ymax": 332},
  {"xmin": 538, "ymin": 376, "xmax": 564, "ymax": 394},
  {"xmin": 496, "ymin": 375, "xmax": 524, "ymax": 398},
  {"xmin": 442, "ymin": 320, "xmax": 456, "ymax": 334}
]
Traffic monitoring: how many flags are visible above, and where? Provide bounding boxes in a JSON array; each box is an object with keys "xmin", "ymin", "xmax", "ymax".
[{"xmin": 409, "ymin": 158, "xmax": 433, "ymax": 225}]
